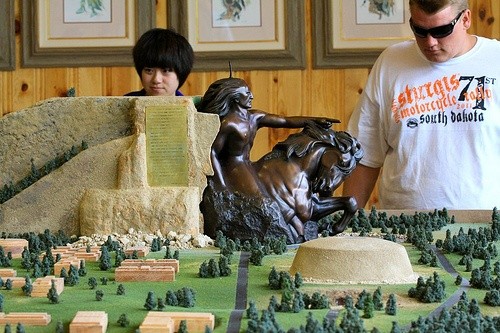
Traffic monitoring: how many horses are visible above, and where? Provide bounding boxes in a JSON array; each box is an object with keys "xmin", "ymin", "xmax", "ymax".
[{"xmin": 198, "ymin": 121, "xmax": 364, "ymax": 241}]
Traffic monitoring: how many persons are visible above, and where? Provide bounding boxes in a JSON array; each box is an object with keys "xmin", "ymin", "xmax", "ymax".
[
  {"xmin": 123, "ymin": 28, "xmax": 194, "ymax": 96},
  {"xmin": 196, "ymin": 78, "xmax": 341, "ymax": 197},
  {"xmin": 344, "ymin": 0, "xmax": 499, "ymax": 212}
]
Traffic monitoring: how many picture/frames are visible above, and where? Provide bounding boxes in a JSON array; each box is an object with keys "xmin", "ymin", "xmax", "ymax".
[
  {"xmin": 166, "ymin": 0, "xmax": 307, "ymax": 70},
  {"xmin": 311, "ymin": 0, "xmax": 416, "ymax": 69},
  {"xmin": 19, "ymin": 0, "xmax": 157, "ymax": 66},
  {"xmin": 0, "ymin": 0, "xmax": 16, "ymax": 70}
]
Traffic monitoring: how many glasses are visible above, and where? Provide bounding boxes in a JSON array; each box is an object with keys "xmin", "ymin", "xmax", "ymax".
[{"xmin": 409, "ymin": 9, "xmax": 466, "ymax": 38}]
